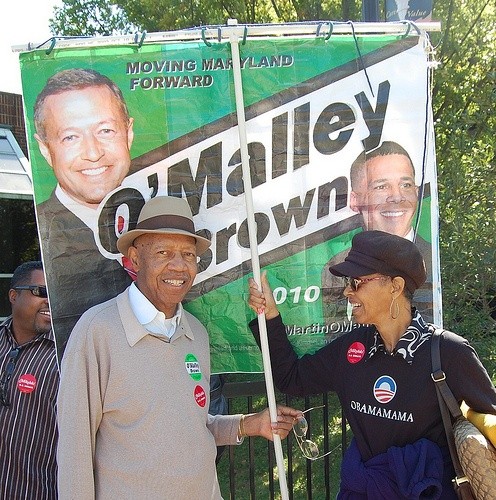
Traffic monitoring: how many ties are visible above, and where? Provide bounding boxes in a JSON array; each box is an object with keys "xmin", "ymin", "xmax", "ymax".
[{"xmin": 113, "ymin": 216, "xmax": 138, "ymax": 283}]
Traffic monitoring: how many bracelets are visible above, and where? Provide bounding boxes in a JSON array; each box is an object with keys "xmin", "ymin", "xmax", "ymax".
[
  {"xmin": 239, "ymin": 425, "xmax": 243, "ymax": 437},
  {"xmin": 242, "ymin": 415, "xmax": 247, "ymax": 437}
]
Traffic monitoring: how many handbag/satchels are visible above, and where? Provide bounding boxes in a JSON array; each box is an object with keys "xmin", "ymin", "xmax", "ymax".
[{"xmin": 430, "ymin": 329, "xmax": 496, "ymax": 500}]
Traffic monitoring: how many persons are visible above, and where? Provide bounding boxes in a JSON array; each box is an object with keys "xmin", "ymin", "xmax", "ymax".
[
  {"xmin": 0, "ymin": 262, "xmax": 61, "ymax": 500},
  {"xmin": 248, "ymin": 231, "xmax": 496, "ymax": 500},
  {"xmin": 320, "ymin": 139, "xmax": 433, "ymax": 337},
  {"xmin": 34, "ymin": 68, "xmax": 135, "ymax": 368},
  {"xmin": 55, "ymin": 196, "xmax": 304, "ymax": 500}
]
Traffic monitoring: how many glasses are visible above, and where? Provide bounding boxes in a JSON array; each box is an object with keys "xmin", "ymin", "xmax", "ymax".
[
  {"xmin": 6, "ymin": 350, "xmax": 20, "ymax": 373},
  {"xmin": 14, "ymin": 285, "xmax": 46, "ymax": 298},
  {"xmin": 293, "ymin": 405, "xmax": 342, "ymax": 461},
  {"xmin": 344, "ymin": 277, "xmax": 389, "ymax": 291}
]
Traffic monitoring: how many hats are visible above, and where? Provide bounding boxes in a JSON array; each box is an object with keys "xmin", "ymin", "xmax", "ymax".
[
  {"xmin": 117, "ymin": 196, "xmax": 211, "ymax": 255},
  {"xmin": 329, "ymin": 230, "xmax": 426, "ymax": 292}
]
